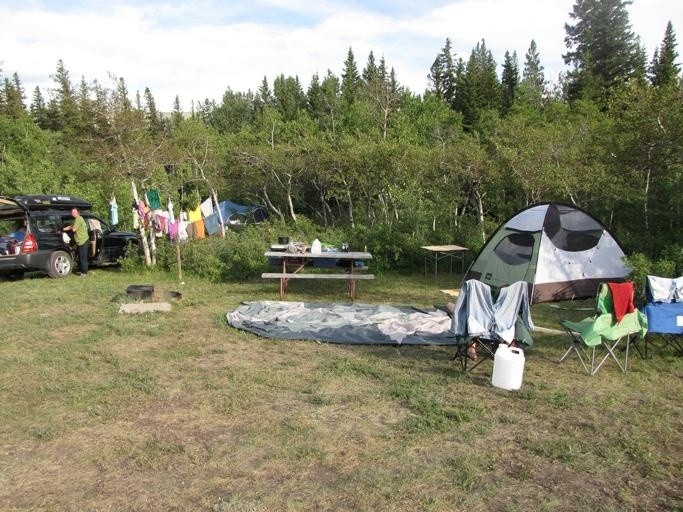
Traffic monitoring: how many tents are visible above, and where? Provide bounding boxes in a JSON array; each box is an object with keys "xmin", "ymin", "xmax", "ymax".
[{"xmin": 461, "ymin": 202, "xmax": 636, "ymax": 305}]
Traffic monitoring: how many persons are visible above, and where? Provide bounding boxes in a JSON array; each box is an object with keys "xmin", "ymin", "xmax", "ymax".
[{"xmin": 63, "ymin": 208, "xmax": 90, "ymax": 277}]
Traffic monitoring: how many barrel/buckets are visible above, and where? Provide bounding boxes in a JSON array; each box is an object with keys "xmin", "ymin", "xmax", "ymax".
[
  {"xmin": 311, "ymin": 238, "xmax": 321, "ymax": 254},
  {"xmin": 492, "ymin": 343, "xmax": 526, "ymax": 391}
]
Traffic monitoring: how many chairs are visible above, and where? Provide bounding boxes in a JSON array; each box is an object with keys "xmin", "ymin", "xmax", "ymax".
[
  {"xmin": 622, "ymin": 274, "xmax": 683, "ymax": 360},
  {"xmin": 449, "ymin": 279, "xmax": 534, "ymax": 374},
  {"xmin": 560, "ymin": 280, "xmax": 648, "ymax": 375}
]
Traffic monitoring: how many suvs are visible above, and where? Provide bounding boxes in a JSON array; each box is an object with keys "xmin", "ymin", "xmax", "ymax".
[{"xmin": 0, "ymin": 192, "xmax": 143, "ymax": 278}]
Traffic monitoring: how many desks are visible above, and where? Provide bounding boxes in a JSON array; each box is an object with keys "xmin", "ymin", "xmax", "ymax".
[
  {"xmin": 420, "ymin": 244, "xmax": 470, "ymax": 281},
  {"xmin": 264, "ymin": 251, "xmax": 372, "ymax": 299}
]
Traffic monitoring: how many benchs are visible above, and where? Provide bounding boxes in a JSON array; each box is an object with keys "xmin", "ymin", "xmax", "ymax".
[{"xmin": 261, "ymin": 273, "xmax": 375, "ymax": 302}]
